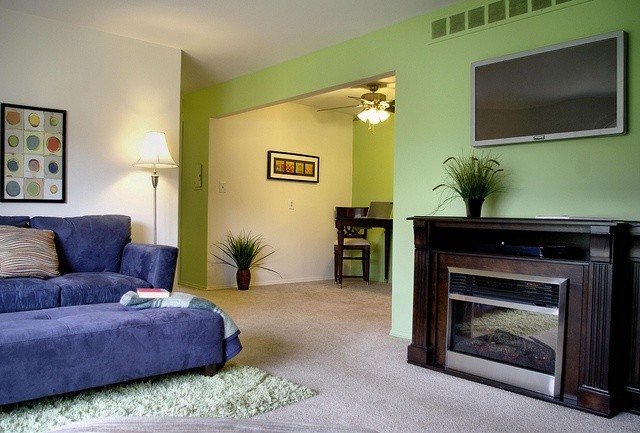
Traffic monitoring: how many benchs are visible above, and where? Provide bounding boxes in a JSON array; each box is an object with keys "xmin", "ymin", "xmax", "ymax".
[{"xmin": 1, "ymin": 299, "xmax": 223, "ymax": 407}]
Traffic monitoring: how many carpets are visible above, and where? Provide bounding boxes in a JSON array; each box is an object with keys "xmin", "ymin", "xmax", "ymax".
[{"xmin": 1, "ymin": 364, "xmax": 316, "ymax": 432}]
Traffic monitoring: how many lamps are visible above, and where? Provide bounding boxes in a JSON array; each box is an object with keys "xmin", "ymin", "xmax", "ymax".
[
  {"xmin": 356, "ymin": 104, "xmax": 390, "ymax": 135},
  {"xmin": 130, "ymin": 130, "xmax": 180, "ymax": 247}
]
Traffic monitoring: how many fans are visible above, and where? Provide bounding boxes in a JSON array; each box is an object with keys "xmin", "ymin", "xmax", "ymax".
[{"xmin": 317, "ymin": 80, "xmax": 395, "ymax": 123}]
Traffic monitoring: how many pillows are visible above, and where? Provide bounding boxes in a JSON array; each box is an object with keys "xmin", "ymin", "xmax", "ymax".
[{"xmin": 0, "ymin": 224, "xmax": 61, "ymax": 280}]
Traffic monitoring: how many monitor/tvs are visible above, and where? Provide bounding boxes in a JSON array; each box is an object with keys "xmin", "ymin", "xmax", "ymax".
[{"xmin": 467, "ymin": 28, "xmax": 628, "ymax": 149}]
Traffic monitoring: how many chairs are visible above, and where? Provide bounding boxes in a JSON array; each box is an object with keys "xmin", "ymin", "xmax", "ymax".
[{"xmin": 333, "ymin": 206, "xmax": 370, "ymax": 286}]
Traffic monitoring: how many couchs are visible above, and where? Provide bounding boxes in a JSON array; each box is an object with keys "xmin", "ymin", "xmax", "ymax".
[{"xmin": 0, "ymin": 215, "xmax": 179, "ymax": 312}]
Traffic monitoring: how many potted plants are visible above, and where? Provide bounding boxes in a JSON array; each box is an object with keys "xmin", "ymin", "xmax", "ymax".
[
  {"xmin": 209, "ymin": 228, "xmax": 284, "ymax": 291},
  {"xmin": 432, "ymin": 147, "xmax": 511, "ymax": 216}
]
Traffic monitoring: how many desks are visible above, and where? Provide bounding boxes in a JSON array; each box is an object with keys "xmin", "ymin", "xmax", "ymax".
[{"xmin": 335, "ymin": 216, "xmax": 393, "ymax": 291}]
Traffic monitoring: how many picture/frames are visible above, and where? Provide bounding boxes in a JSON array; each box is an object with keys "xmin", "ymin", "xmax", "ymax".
[
  {"xmin": 267, "ymin": 150, "xmax": 320, "ymax": 183},
  {"xmin": 1, "ymin": 102, "xmax": 67, "ymax": 204}
]
